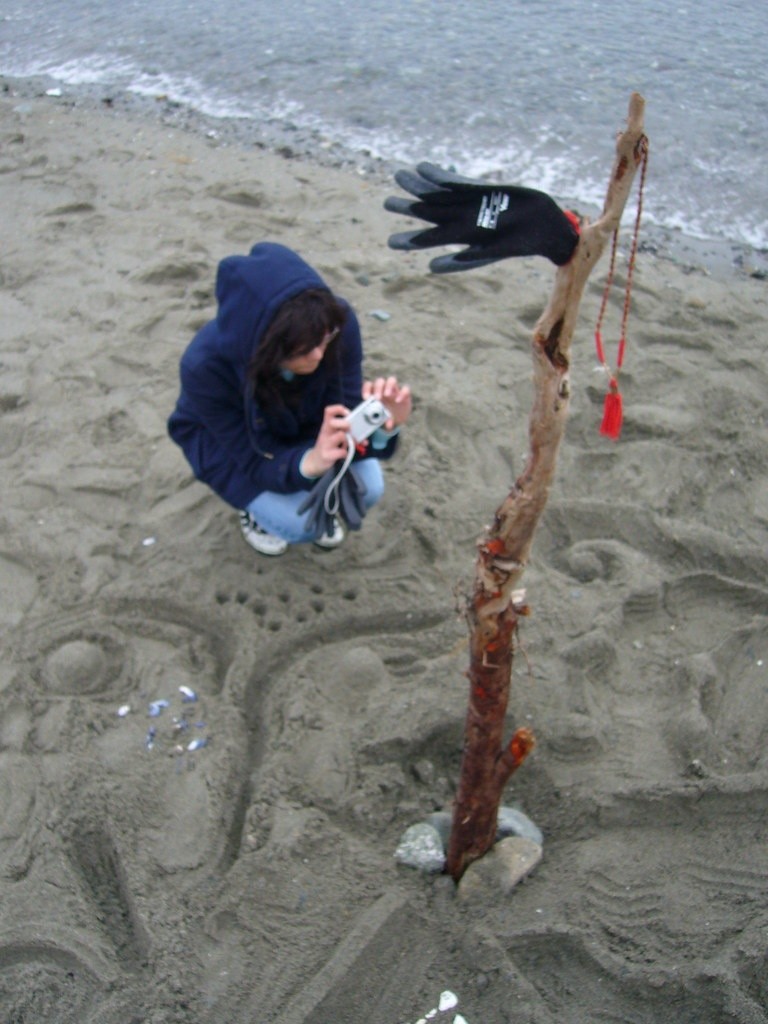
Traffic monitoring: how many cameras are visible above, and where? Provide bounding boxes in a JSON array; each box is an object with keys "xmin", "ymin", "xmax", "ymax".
[{"xmin": 340, "ymin": 396, "xmax": 391, "ymax": 444}]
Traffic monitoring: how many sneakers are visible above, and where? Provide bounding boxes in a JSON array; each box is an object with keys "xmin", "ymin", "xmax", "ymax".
[
  {"xmin": 238, "ymin": 510, "xmax": 288, "ymax": 555},
  {"xmin": 313, "ymin": 519, "xmax": 345, "ymax": 548}
]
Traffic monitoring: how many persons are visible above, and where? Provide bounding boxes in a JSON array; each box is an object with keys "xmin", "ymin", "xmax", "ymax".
[{"xmin": 168, "ymin": 239, "xmax": 415, "ymax": 557}]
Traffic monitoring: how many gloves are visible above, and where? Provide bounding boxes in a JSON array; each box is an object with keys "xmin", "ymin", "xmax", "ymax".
[{"xmin": 384, "ymin": 159, "xmax": 581, "ymax": 274}]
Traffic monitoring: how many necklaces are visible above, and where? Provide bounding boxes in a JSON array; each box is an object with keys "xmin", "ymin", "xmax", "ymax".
[{"xmin": 596, "ymin": 132, "xmax": 649, "ymax": 439}]
